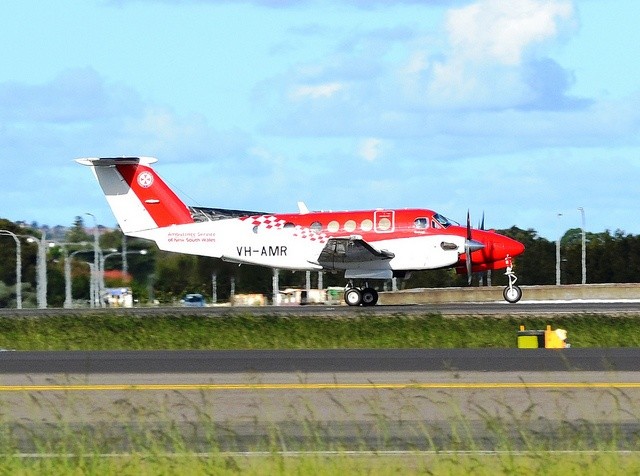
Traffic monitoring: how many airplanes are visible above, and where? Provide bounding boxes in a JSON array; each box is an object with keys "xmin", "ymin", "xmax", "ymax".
[{"xmin": 76, "ymin": 156, "xmax": 525, "ymax": 306}]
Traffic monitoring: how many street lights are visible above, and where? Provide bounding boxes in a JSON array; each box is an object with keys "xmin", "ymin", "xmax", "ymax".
[
  {"xmin": 553, "ymin": 212, "xmax": 564, "ymax": 286},
  {"xmin": 1, "ymin": 211, "xmax": 148, "ymax": 308},
  {"xmin": 576, "ymin": 206, "xmax": 586, "ymax": 285}
]
